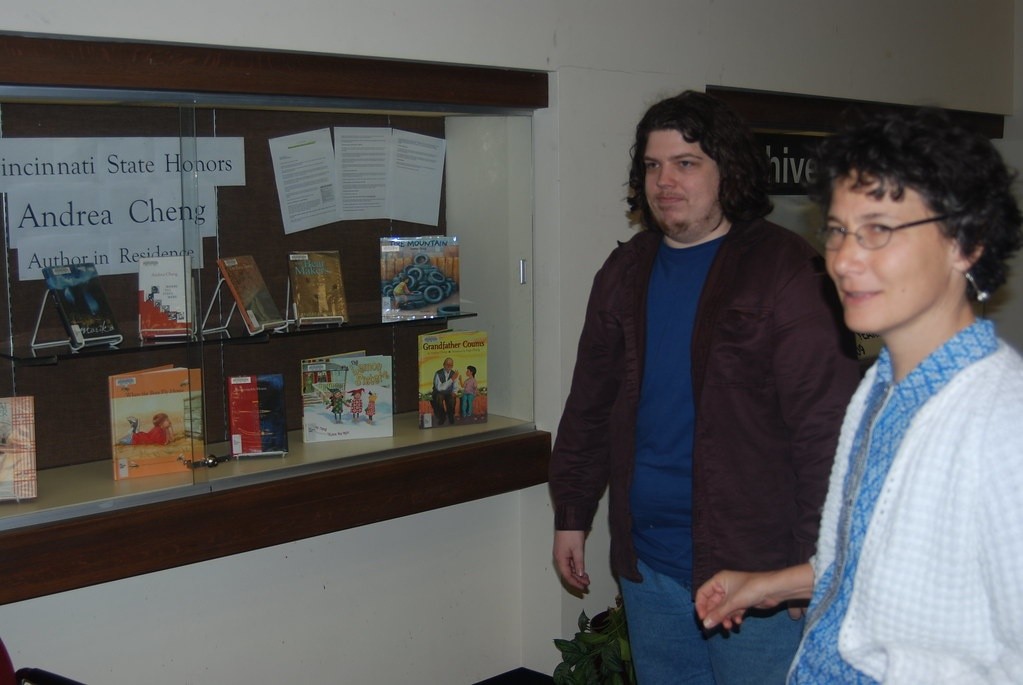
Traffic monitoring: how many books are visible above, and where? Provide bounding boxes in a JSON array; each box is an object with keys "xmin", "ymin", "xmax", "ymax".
[
  {"xmin": 138, "ymin": 255, "xmax": 193, "ymax": 338},
  {"xmin": 416, "ymin": 329, "xmax": 488, "ymax": 429},
  {"xmin": 108, "ymin": 364, "xmax": 205, "ymax": 481},
  {"xmin": 41, "ymin": 263, "xmax": 125, "ymax": 348},
  {"xmin": 227, "ymin": 373, "xmax": 289, "ymax": 457},
  {"xmin": 0, "ymin": 395, "xmax": 37, "ymax": 500},
  {"xmin": 286, "ymin": 250, "xmax": 351, "ymax": 324},
  {"xmin": 216, "ymin": 255, "xmax": 285, "ymax": 333},
  {"xmin": 301, "ymin": 350, "xmax": 393, "ymax": 443},
  {"xmin": 378, "ymin": 235, "xmax": 460, "ymax": 324}
]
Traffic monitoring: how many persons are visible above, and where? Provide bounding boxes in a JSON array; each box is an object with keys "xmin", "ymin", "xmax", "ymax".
[
  {"xmin": 548, "ymin": 89, "xmax": 862, "ymax": 685},
  {"xmin": 694, "ymin": 103, "xmax": 1023, "ymax": 685}
]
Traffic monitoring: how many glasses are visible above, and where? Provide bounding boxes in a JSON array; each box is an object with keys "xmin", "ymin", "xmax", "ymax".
[{"xmin": 816, "ymin": 217, "xmax": 945, "ymax": 249}]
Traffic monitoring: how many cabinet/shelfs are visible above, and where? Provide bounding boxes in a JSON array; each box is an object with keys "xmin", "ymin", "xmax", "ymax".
[{"xmin": 0, "ymin": 30, "xmax": 552, "ymax": 605}]
